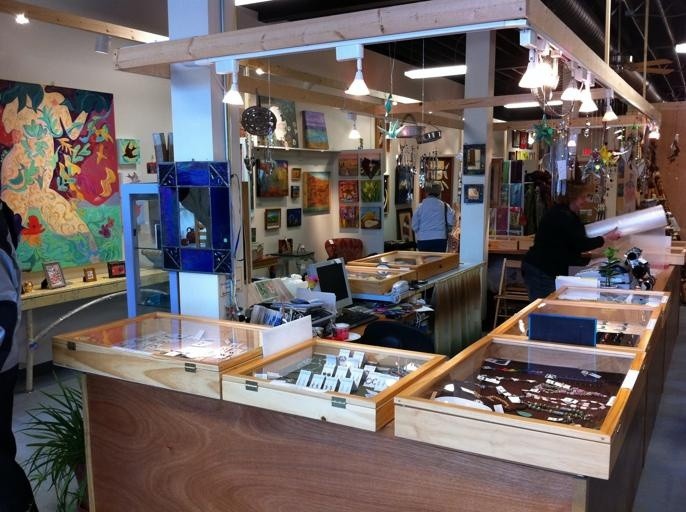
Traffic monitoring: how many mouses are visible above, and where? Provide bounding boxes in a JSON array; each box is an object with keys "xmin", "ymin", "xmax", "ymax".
[{"xmin": 386, "ymin": 312, "xmax": 400, "ymax": 320}]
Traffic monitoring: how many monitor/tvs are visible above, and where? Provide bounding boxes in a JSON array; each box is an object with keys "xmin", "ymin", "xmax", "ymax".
[{"xmin": 308, "ymin": 257, "xmax": 353, "ymax": 308}]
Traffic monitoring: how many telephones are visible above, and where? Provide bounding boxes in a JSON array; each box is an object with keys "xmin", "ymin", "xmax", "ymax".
[{"xmin": 392, "ymin": 280, "xmax": 409, "ymax": 294}]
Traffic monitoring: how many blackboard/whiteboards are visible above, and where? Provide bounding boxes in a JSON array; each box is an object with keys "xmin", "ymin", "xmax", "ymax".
[
  {"xmin": 0, "ymin": 80, "xmax": 123, "ymax": 272},
  {"xmin": 257, "ymin": 96, "xmax": 299, "ymax": 147}
]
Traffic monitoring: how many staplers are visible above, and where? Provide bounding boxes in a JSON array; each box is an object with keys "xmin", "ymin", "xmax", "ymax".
[{"xmin": 260, "ymin": 303, "xmax": 273, "ymax": 308}]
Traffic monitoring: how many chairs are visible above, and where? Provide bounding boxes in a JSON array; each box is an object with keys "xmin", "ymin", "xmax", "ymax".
[
  {"xmin": 492, "ymin": 257, "xmax": 530, "ymax": 328},
  {"xmin": 325, "ymin": 237, "xmax": 378, "ymax": 261}
]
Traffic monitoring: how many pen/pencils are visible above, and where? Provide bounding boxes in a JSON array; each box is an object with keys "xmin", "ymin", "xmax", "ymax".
[
  {"xmin": 280, "ymin": 305, "xmax": 299, "ymax": 322},
  {"xmin": 331, "ymin": 323, "xmax": 337, "ymax": 328}
]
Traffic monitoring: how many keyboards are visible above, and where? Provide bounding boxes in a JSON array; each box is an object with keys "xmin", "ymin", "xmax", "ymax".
[{"xmin": 336, "ymin": 311, "xmax": 379, "ymax": 327}]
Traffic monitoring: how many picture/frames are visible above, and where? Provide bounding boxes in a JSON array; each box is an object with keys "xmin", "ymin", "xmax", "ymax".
[
  {"xmin": 105, "ymin": 259, "xmax": 126, "ymax": 279},
  {"xmin": 286, "ymin": 207, "xmax": 302, "ymax": 228},
  {"xmin": 40, "ymin": 261, "xmax": 67, "ymax": 291},
  {"xmin": 395, "ymin": 206, "xmax": 417, "ymax": 247},
  {"xmin": 264, "ymin": 208, "xmax": 281, "ymax": 230},
  {"xmin": 463, "ymin": 183, "xmax": 484, "ymax": 205},
  {"xmin": 83, "ymin": 265, "xmax": 96, "ymax": 283}
]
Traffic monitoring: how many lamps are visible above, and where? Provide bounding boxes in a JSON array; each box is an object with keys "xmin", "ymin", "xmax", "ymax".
[
  {"xmin": 600, "ymin": 96, "xmax": 619, "ymax": 122},
  {"xmin": 402, "ymin": 32, "xmax": 468, "ymax": 80},
  {"xmin": 560, "ymin": 66, "xmax": 585, "ymax": 103},
  {"xmin": 346, "ymin": 110, "xmax": 361, "ymax": 141},
  {"xmin": 576, "ymin": 67, "xmax": 600, "ymax": 114},
  {"xmin": 516, "ymin": 48, "xmax": 559, "ymax": 89},
  {"xmin": 93, "ymin": 34, "xmax": 113, "ymax": 54},
  {"xmin": 331, "ymin": 42, "xmax": 370, "ymax": 98},
  {"xmin": 212, "ymin": 57, "xmax": 245, "ymax": 109}
]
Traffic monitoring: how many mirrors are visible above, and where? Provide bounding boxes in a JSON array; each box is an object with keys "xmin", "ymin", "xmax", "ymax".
[
  {"xmin": 155, "ymin": 158, "xmax": 234, "ymax": 277},
  {"xmin": 462, "ymin": 142, "xmax": 486, "ymax": 176}
]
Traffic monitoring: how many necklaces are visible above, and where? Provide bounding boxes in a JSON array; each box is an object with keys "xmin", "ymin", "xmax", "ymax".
[{"xmin": 516, "ymin": 378, "xmax": 611, "ymax": 422}]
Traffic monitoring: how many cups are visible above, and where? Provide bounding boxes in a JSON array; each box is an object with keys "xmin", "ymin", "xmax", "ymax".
[
  {"xmin": 332, "ymin": 322, "xmax": 350, "ymax": 339},
  {"xmin": 312, "ymin": 327, "xmax": 324, "ymax": 337}
]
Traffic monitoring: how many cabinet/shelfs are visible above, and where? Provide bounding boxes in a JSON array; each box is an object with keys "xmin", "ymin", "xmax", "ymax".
[{"xmin": 119, "ymin": 181, "xmax": 179, "ymax": 319}]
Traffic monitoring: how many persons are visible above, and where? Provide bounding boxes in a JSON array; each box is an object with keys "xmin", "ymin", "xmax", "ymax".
[
  {"xmin": 0, "ymin": 199, "xmax": 40, "ymax": 511},
  {"xmin": 413, "ymin": 181, "xmax": 455, "ymax": 251},
  {"xmin": 520, "ymin": 179, "xmax": 622, "ymax": 302}
]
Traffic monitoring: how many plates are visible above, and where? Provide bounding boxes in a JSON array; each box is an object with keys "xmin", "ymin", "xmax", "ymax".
[{"xmin": 328, "ymin": 332, "xmax": 361, "ymax": 342}]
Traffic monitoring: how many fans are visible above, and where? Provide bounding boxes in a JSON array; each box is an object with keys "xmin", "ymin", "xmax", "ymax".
[{"xmin": 605, "ymin": 0, "xmax": 674, "ymax": 79}]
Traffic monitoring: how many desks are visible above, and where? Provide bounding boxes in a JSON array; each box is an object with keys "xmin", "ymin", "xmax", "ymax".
[
  {"xmin": 267, "ymin": 250, "xmax": 316, "ymax": 277},
  {"xmin": 251, "ymin": 260, "xmax": 285, "ymax": 279},
  {"xmin": 19, "ymin": 265, "xmax": 170, "ymax": 395},
  {"xmin": 75, "ymin": 267, "xmax": 685, "ymax": 512},
  {"xmin": 383, "ymin": 240, "xmax": 410, "ymax": 253}
]
